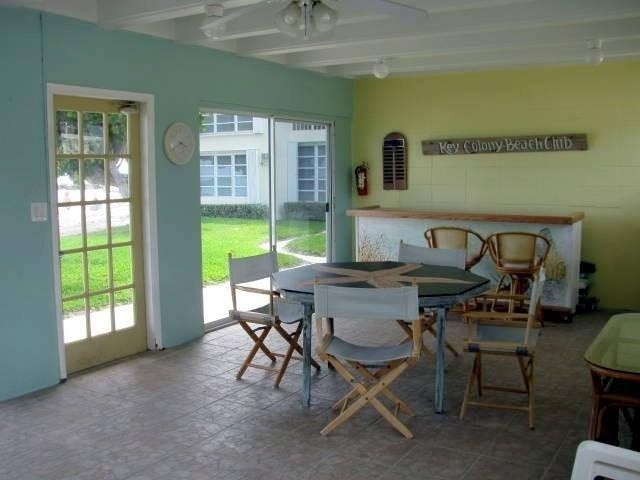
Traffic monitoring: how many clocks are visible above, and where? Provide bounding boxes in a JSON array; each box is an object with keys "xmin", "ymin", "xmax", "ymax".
[{"xmin": 163, "ymin": 121, "xmax": 195, "ymax": 166}]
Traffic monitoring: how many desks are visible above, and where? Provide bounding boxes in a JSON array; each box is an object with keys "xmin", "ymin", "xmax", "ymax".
[{"xmin": 585, "ymin": 310, "xmax": 640, "ymax": 442}]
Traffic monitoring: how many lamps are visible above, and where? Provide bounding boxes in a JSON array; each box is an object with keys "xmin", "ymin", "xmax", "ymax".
[
  {"xmin": 371, "ymin": 57, "xmax": 390, "ymax": 80},
  {"xmin": 200, "ymin": 5, "xmax": 227, "ymax": 38},
  {"xmin": 583, "ymin": 40, "xmax": 604, "ymax": 65},
  {"xmin": 274, "ymin": 0, "xmax": 340, "ymax": 43}
]
